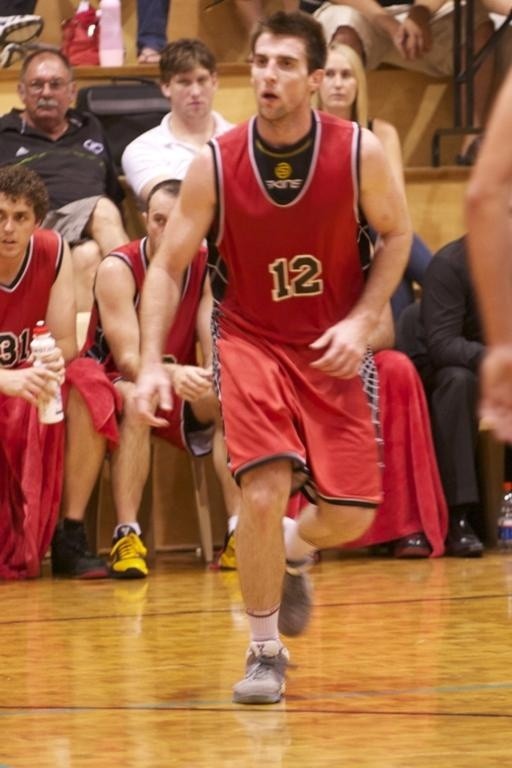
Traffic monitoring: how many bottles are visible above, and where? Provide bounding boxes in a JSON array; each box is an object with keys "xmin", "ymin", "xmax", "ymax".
[
  {"xmin": 496, "ymin": 482, "xmax": 512, "ymax": 555},
  {"xmin": 95, "ymin": 0, "xmax": 124, "ymax": 68},
  {"xmin": 30, "ymin": 327, "xmax": 64, "ymax": 425}
]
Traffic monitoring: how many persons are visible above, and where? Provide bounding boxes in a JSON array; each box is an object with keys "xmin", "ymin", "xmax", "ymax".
[
  {"xmin": 0, "ymin": 48, "xmax": 133, "ymax": 357},
  {"xmin": 316, "ymin": 41, "xmax": 403, "ymax": 203},
  {"xmin": 0, "ymin": 0, "xmax": 512, "ymax": 172},
  {"xmin": 0, "ymin": 164, "xmax": 123, "ymax": 582},
  {"xmin": 135, "ymin": 11, "xmax": 415, "ymax": 706},
  {"xmin": 78, "ymin": 179, "xmax": 244, "ymax": 581},
  {"xmin": 285, "ymin": 237, "xmax": 490, "ymax": 558},
  {"xmin": 120, "ymin": 38, "xmax": 241, "ymax": 205},
  {"xmin": 464, "ymin": 66, "xmax": 511, "ymax": 442}
]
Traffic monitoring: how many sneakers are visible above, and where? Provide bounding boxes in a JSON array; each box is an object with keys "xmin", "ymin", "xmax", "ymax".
[
  {"xmin": 110, "ymin": 522, "xmax": 149, "ymax": 578},
  {"xmin": 233, "ymin": 640, "xmax": 289, "ymax": 705},
  {"xmin": 447, "ymin": 519, "xmax": 485, "ymax": 557},
  {"xmin": 278, "ymin": 558, "xmax": 315, "ymax": 636},
  {"xmin": 217, "ymin": 529, "xmax": 237, "ymax": 569},
  {"xmin": 51, "ymin": 531, "xmax": 108, "ymax": 580},
  {"xmin": 394, "ymin": 532, "xmax": 430, "ymax": 558}
]
